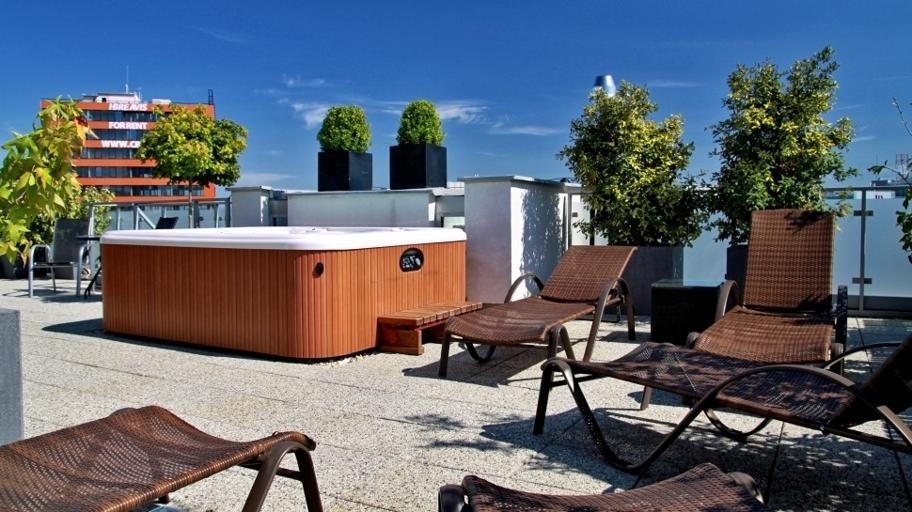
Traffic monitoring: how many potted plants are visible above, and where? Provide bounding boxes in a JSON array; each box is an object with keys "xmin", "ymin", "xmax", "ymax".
[
  {"xmin": 712, "ymin": 42, "xmax": 852, "ymax": 306},
  {"xmin": 316, "ymin": 105, "xmax": 373, "ymax": 192},
  {"xmin": 388, "ymin": 100, "xmax": 447, "ymax": 189},
  {"xmin": 556, "ymin": 82, "xmax": 707, "ymax": 312}
]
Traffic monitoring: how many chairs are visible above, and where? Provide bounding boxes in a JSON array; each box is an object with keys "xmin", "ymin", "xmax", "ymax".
[
  {"xmin": 27, "ymin": 217, "xmax": 90, "ymax": 295},
  {"xmin": 437, "ymin": 464, "xmax": 768, "ymax": 512},
  {"xmin": 1, "ymin": 404, "xmax": 320, "ymax": 511},
  {"xmin": 86, "ymin": 216, "xmax": 178, "ymax": 296},
  {"xmin": 534, "ymin": 320, "xmax": 911, "ymax": 476},
  {"xmin": 687, "ymin": 208, "xmax": 848, "ymax": 384},
  {"xmin": 440, "ymin": 243, "xmax": 637, "ymax": 381}
]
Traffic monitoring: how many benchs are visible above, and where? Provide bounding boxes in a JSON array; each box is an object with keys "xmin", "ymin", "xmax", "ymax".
[{"xmin": 378, "ymin": 301, "xmax": 482, "ymax": 356}]
